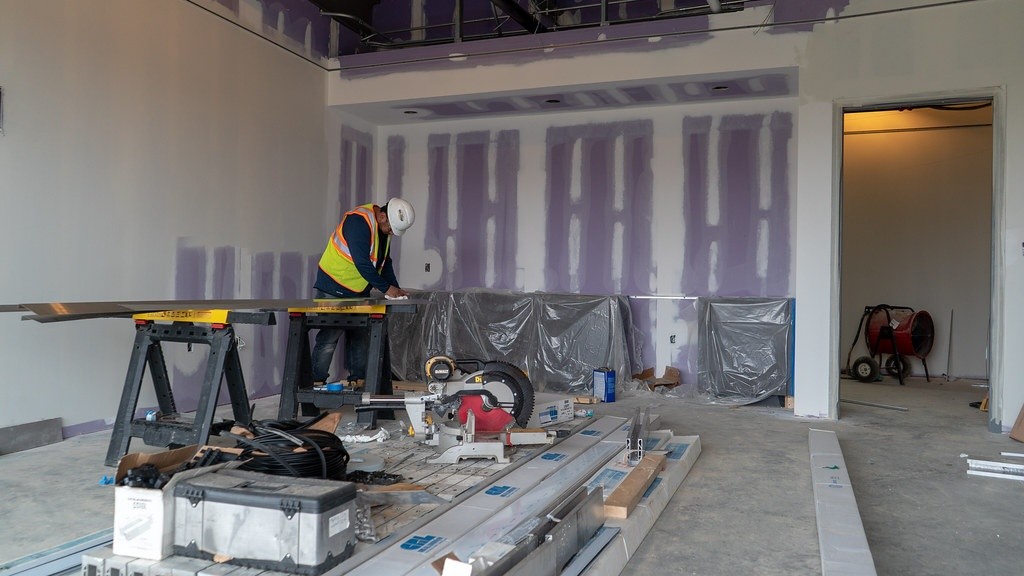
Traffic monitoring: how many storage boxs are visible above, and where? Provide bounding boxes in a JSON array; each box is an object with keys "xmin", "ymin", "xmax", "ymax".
[
  {"xmin": 111, "ymin": 442, "xmax": 255, "ymax": 561},
  {"xmin": 173, "ymin": 467, "xmax": 358, "ymax": 576}
]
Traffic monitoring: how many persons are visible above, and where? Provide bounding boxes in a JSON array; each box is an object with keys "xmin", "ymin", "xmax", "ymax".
[{"xmin": 312, "ymin": 197, "xmax": 415, "ymax": 390}]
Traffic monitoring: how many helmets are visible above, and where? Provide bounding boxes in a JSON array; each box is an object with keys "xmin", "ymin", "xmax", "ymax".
[{"xmin": 387, "ymin": 198, "xmax": 415, "ymax": 236}]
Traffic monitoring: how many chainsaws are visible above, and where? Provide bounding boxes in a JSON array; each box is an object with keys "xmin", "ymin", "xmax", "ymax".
[{"xmin": 424, "ymin": 354, "xmax": 571, "ymax": 464}]
